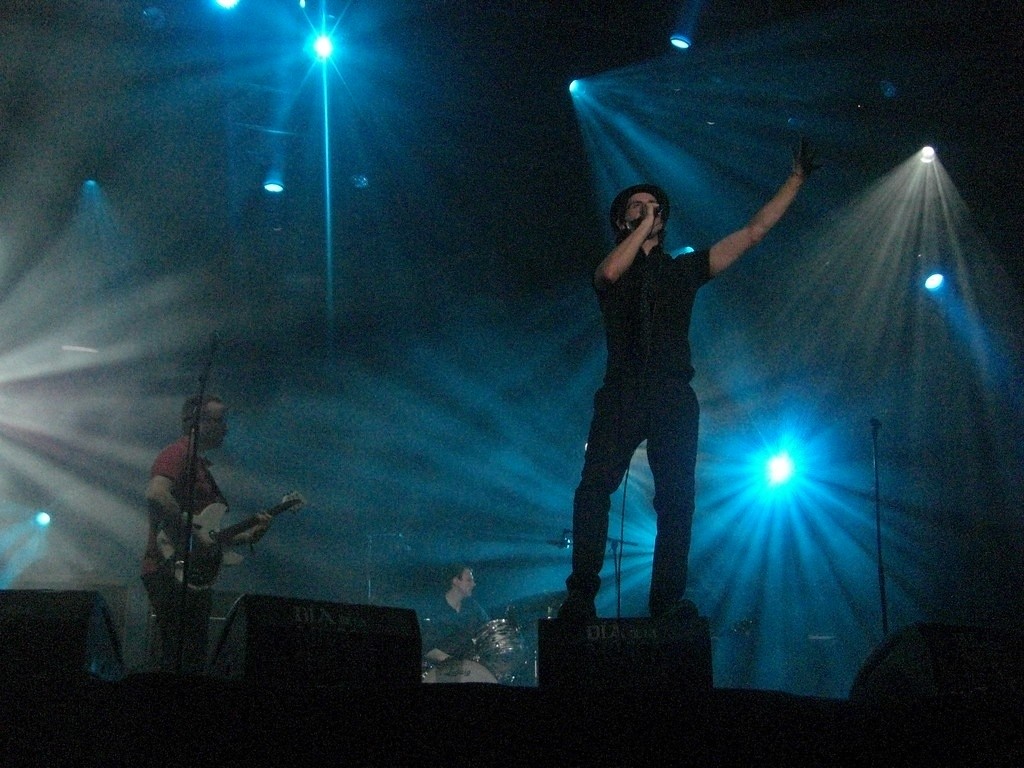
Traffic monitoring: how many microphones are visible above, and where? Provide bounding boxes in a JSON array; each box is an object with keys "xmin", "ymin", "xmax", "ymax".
[
  {"xmin": 653, "ymin": 202, "xmax": 667, "ymax": 217},
  {"xmin": 544, "ymin": 537, "xmax": 571, "ymax": 547}
]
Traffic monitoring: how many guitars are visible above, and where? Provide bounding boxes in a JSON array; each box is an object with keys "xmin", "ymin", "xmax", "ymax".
[{"xmin": 154, "ymin": 490, "xmax": 309, "ymax": 593}]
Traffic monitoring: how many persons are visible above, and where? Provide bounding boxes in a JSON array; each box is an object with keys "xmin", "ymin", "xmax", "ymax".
[
  {"xmin": 416, "ymin": 557, "xmax": 498, "ymax": 681},
  {"xmin": 139, "ymin": 390, "xmax": 279, "ymax": 688},
  {"xmin": 548, "ymin": 133, "xmax": 827, "ymax": 631}
]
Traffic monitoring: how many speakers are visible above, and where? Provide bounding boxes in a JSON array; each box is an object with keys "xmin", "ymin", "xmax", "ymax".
[
  {"xmin": 851, "ymin": 621, "xmax": 1024, "ymax": 713},
  {"xmin": 0, "ymin": 590, "xmax": 126, "ymax": 676},
  {"xmin": 203, "ymin": 594, "xmax": 423, "ymax": 683},
  {"xmin": 540, "ymin": 618, "xmax": 716, "ymax": 695}
]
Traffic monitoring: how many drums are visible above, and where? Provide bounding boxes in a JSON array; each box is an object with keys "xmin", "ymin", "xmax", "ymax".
[
  {"xmin": 422, "ymin": 655, "xmax": 498, "ymax": 683},
  {"xmin": 473, "ymin": 618, "xmax": 528, "ymax": 681}
]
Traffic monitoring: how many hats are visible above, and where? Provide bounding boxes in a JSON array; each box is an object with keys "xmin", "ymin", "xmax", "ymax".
[{"xmin": 610, "ymin": 183, "xmax": 670, "ymax": 237}]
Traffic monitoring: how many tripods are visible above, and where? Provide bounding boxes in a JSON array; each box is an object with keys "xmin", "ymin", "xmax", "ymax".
[{"xmin": 126, "ymin": 327, "xmax": 223, "ymax": 678}]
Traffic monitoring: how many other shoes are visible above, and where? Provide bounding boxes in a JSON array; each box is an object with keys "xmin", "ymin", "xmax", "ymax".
[{"xmin": 562, "ymin": 600, "xmax": 697, "ymax": 620}]
